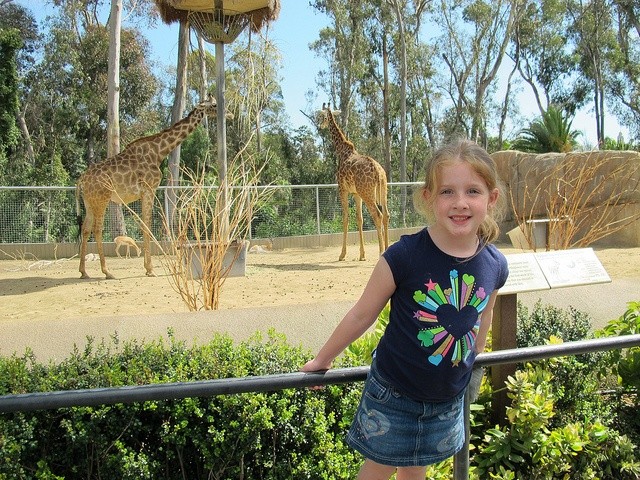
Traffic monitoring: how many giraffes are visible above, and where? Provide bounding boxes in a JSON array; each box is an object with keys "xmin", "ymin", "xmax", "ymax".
[
  {"xmin": 75, "ymin": 92, "xmax": 235, "ymax": 279},
  {"xmin": 317, "ymin": 102, "xmax": 390, "ymax": 261},
  {"xmin": 114, "ymin": 236, "xmax": 142, "ymax": 260}
]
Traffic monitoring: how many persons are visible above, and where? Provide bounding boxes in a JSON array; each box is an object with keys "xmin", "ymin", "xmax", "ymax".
[{"xmin": 300, "ymin": 138, "xmax": 509, "ymax": 480}]
